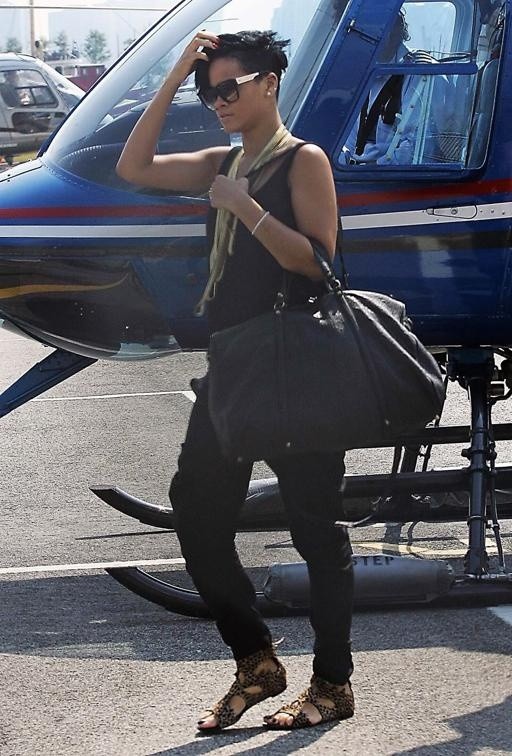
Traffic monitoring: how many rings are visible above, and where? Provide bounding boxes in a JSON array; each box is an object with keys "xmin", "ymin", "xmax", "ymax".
[{"xmin": 207, "ymin": 188, "xmax": 214, "ymax": 200}]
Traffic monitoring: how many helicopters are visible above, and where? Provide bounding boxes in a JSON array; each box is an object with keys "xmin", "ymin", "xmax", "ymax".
[
  {"xmin": 0, "ymin": 50, "xmax": 114, "ymax": 172},
  {"xmin": 0, "ymin": 0, "xmax": 512, "ymax": 620}
]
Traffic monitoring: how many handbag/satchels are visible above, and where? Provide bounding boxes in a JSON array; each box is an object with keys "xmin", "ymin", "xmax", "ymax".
[{"xmin": 200, "ymin": 234, "xmax": 449, "ymax": 476}]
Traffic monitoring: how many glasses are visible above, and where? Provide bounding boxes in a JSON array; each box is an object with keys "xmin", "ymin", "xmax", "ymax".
[{"xmin": 195, "ymin": 69, "xmax": 267, "ymax": 112}]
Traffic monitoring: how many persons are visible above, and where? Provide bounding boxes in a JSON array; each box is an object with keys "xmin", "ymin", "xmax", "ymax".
[
  {"xmin": 113, "ymin": 29, "xmax": 356, "ymax": 736},
  {"xmin": 331, "ymin": 1, "xmax": 460, "ymax": 166}
]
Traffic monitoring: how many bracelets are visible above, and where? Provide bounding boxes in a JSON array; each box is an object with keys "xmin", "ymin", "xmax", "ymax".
[{"xmin": 250, "ymin": 210, "xmax": 270, "ymax": 236}]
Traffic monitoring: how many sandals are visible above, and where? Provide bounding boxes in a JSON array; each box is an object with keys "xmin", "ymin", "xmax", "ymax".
[
  {"xmin": 196, "ymin": 651, "xmax": 288, "ymax": 735},
  {"xmin": 261, "ymin": 667, "xmax": 356, "ymax": 735}
]
